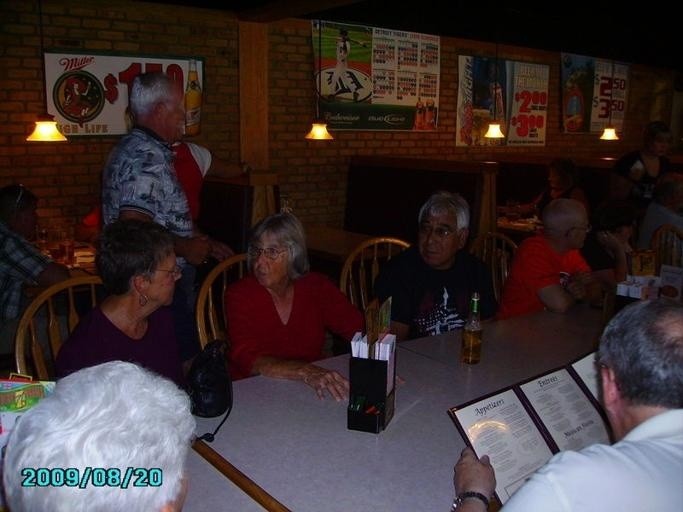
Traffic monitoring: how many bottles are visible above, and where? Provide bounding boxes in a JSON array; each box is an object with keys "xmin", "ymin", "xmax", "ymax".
[
  {"xmin": 462, "ymin": 291, "xmax": 484, "ymax": 365},
  {"xmin": 184, "ymin": 58, "xmax": 201, "ymax": 136}
]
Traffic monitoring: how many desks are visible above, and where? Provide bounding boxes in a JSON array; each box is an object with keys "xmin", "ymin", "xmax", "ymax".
[{"xmin": 296, "ymin": 220, "xmax": 412, "ymax": 266}]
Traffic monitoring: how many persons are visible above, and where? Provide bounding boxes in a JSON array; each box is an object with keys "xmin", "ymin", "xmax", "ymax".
[
  {"xmin": 452, "ymin": 300, "xmax": 683, "ymax": 512},
  {"xmin": 372, "ymin": 192, "xmax": 495, "ymax": 340},
  {"xmin": 225, "ymin": 211, "xmax": 406, "ymax": 401},
  {"xmin": 0, "ymin": 185, "xmax": 58, "ymax": 357},
  {"xmin": 3, "ymin": 360, "xmax": 196, "ymax": 511},
  {"xmin": 100, "ymin": 72, "xmax": 215, "ymax": 305},
  {"xmin": 496, "ymin": 197, "xmax": 596, "ymax": 316},
  {"xmin": 170, "ymin": 142, "xmax": 250, "ymax": 223},
  {"xmin": 510, "ymin": 159, "xmax": 587, "ymax": 217},
  {"xmin": 52, "ymin": 218, "xmax": 202, "ymax": 386},
  {"xmin": 639, "ymin": 172, "xmax": 683, "ymax": 254},
  {"xmin": 612, "ymin": 121, "xmax": 677, "ymax": 189}
]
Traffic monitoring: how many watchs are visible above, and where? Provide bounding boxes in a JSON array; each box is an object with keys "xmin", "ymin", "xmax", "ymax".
[{"xmin": 450, "ymin": 491, "xmax": 490, "ymax": 510}]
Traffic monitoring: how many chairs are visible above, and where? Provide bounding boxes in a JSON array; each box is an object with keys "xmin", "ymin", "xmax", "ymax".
[
  {"xmin": 339, "ymin": 235, "xmax": 411, "ymax": 316},
  {"xmin": 345, "ymin": 153, "xmax": 513, "ymax": 271},
  {"xmin": 498, "ymin": 156, "xmax": 607, "ymax": 209},
  {"xmin": 648, "ymin": 223, "xmax": 683, "ymax": 278},
  {"xmin": 195, "ymin": 252, "xmax": 251, "ymax": 351},
  {"xmin": 15, "ymin": 275, "xmax": 106, "ymax": 381},
  {"xmin": 469, "ymin": 230, "xmax": 518, "ymax": 301},
  {"xmin": 196, "ymin": 175, "xmax": 282, "ymax": 279}
]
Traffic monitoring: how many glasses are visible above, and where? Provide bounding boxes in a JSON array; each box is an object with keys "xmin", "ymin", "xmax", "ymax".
[
  {"xmin": 146, "ymin": 262, "xmax": 184, "ymax": 275},
  {"xmin": 420, "ymin": 224, "xmax": 458, "ymax": 238},
  {"xmin": 571, "ymin": 223, "xmax": 593, "ymax": 232},
  {"xmin": 247, "ymin": 245, "xmax": 291, "ymax": 260}
]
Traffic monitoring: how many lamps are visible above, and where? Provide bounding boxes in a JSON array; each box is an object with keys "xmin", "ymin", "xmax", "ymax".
[
  {"xmin": 24, "ymin": 0, "xmax": 67, "ymax": 144},
  {"xmin": 480, "ymin": 42, "xmax": 506, "ymax": 139},
  {"xmin": 599, "ymin": 45, "xmax": 619, "ymax": 141},
  {"xmin": 305, "ymin": 11, "xmax": 333, "ymax": 141}
]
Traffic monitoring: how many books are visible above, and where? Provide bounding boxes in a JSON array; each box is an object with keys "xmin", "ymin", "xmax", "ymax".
[{"xmin": 447, "ymin": 364, "xmax": 612, "ymax": 505}]
